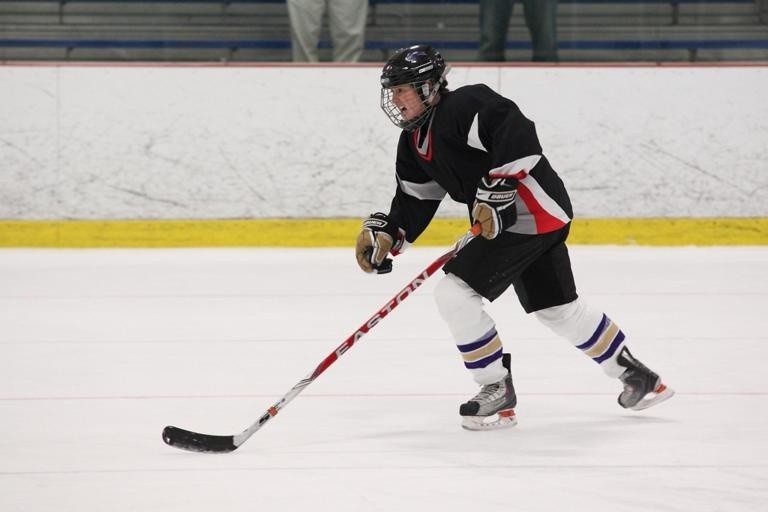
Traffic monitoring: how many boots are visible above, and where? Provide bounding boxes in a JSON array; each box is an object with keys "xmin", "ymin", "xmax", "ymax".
[
  {"xmin": 618, "ymin": 346, "xmax": 661, "ymax": 408},
  {"xmin": 460, "ymin": 354, "xmax": 516, "ymax": 416}
]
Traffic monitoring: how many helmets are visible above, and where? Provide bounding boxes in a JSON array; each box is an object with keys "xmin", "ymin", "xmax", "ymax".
[{"xmin": 381, "ymin": 45, "xmax": 451, "ymax": 133}]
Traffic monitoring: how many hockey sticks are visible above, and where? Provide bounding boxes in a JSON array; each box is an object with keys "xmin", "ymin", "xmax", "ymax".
[{"xmin": 163, "ymin": 221, "xmax": 483, "ymax": 452}]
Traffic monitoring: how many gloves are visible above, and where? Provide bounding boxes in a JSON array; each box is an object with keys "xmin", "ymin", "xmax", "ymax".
[
  {"xmin": 356, "ymin": 213, "xmax": 406, "ymax": 274},
  {"xmin": 472, "ymin": 177, "xmax": 517, "ymax": 239}
]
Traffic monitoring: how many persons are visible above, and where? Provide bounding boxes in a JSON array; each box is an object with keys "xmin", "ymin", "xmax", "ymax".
[
  {"xmin": 353, "ymin": 44, "xmax": 661, "ymax": 416},
  {"xmin": 286, "ymin": 0, "xmax": 369, "ymax": 64},
  {"xmin": 479, "ymin": 0, "xmax": 556, "ymax": 62}
]
